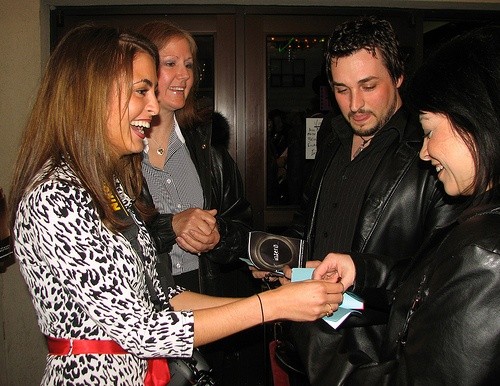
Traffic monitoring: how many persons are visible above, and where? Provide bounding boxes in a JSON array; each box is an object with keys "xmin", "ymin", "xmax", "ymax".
[
  {"xmin": 6, "ymin": 23, "xmax": 344, "ymax": 386},
  {"xmin": 135, "ymin": 15, "xmax": 500, "ymax": 386}
]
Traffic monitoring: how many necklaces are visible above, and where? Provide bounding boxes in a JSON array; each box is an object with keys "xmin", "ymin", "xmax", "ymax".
[
  {"xmin": 147, "ymin": 131, "xmax": 165, "ymax": 156},
  {"xmin": 353, "ymin": 135, "xmax": 371, "ymax": 157}
]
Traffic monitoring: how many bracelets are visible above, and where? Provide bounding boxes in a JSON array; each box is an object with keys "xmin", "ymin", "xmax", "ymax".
[{"xmin": 256, "ymin": 293, "xmax": 265, "ymax": 325}]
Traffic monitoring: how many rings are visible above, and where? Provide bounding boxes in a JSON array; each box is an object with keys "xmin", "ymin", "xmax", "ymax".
[{"xmin": 326, "ymin": 304, "xmax": 334, "ymax": 316}]
